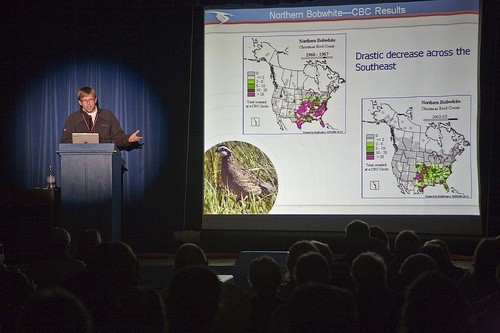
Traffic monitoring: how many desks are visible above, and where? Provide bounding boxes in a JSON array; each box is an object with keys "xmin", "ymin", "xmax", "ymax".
[{"xmin": 0, "ymin": 186, "xmax": 60, "ymax": 253}]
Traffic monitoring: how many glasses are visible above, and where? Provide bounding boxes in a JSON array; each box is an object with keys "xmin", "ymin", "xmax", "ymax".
[{"xmin": 80, "ymin": 99, "xmax": 95, "ymax": 102}]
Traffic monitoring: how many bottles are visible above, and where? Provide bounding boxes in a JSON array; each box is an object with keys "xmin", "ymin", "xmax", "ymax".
[{"xmin": 46, "ymin": 165, "xmax": 56, "ymax": 189}]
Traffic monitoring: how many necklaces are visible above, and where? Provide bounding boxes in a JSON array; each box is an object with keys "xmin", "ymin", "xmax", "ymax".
[{"xmin": 81, "ymin": 113, "xmax": 100, "ymax": 133}]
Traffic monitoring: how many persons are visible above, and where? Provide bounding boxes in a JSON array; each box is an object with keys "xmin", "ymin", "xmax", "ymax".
[
  {"xmin": 0, "ymin": 218, "xmax": 500, "ymax": 333},
  {"xmin": 61, "ymin": 86, "xmax": 144, "ymax": 149}
]
